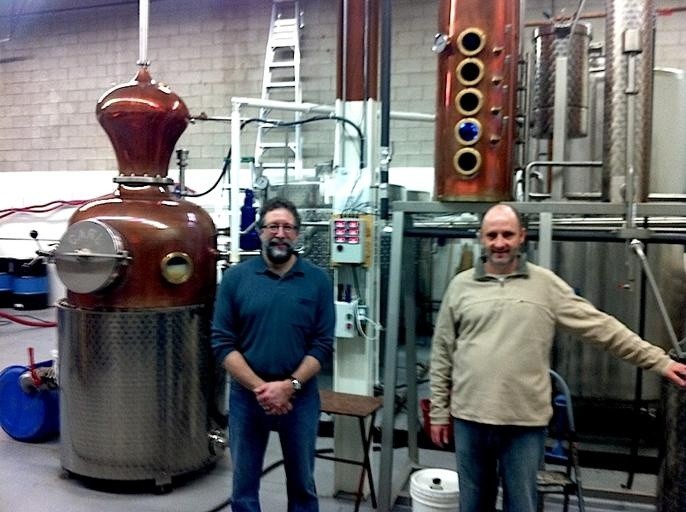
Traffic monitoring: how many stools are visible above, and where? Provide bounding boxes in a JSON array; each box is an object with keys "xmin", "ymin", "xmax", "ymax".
[{"xmin": 319, "ymin": 390, "xmax": 384, "ymax": 511}]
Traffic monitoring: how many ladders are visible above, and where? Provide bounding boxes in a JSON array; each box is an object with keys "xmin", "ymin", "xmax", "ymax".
[{"xmin": 253, "ymin": 0, "xmax": 302, "ymax": 183}]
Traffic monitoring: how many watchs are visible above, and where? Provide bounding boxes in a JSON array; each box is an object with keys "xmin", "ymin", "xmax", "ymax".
[{"xmin": 288, "ymin": 376, "xmax": 303, "ymax": 391}]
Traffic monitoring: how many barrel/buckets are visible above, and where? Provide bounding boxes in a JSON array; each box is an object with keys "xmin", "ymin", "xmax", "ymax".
[
  {"xmin": 409, "ymin": 468, "xmax": 460, "ymax": 511},
  {"xmin": 0, "ymin": 359, "xmax": 59, "ymax": 443},
  {"xmin": 0, "ymin": 257, "xmax": 14, "ymax": 309},
  {"xmin": 13, "ymin": 257, "xmax": 48, "ymax": 310}
]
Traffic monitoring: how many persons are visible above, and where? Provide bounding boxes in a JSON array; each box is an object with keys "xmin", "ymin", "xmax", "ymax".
[
  {"xmin": 429, "ymin": 204, "xmax": 686, "ymax": 512},
  {"xmin": 211, "ymin": 197, "xmax": 335, "ymax": 512}
]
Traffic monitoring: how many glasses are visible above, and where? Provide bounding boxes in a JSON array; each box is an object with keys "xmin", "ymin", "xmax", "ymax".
[{"xmin": 261, "ymin": 222, "xmax": 297, "ymax": 233}]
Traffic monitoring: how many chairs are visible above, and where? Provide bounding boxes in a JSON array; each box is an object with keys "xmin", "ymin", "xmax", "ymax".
[{"xmin": 536, "ymin": 369, "xmax": 587, "ymax": 512}]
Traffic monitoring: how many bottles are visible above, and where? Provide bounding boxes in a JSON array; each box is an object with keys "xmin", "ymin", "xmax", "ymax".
[{"xmin": 430, "ymin": 479, "xmax": 444, "ymax": 492}]
